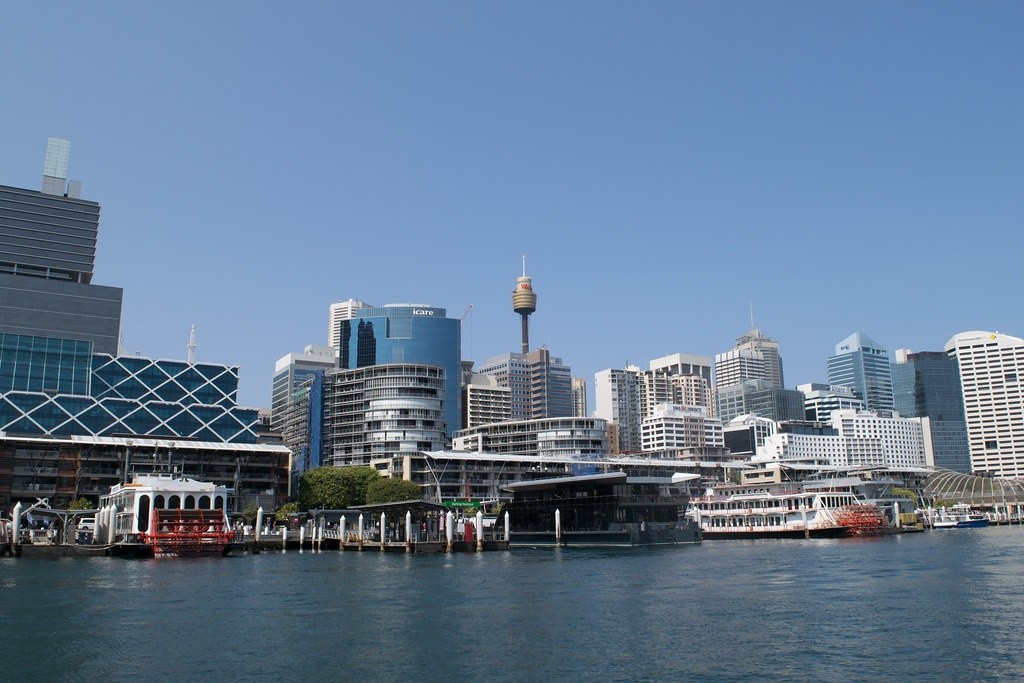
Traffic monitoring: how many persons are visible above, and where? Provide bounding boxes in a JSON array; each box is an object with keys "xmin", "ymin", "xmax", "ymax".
[
  {"xmin": 236, "ymin": 516, "xmax": 246, "ymax": 541},
  {"xmin": 77, "ymin": 526, "xmax": 93, "ymax": 544}
]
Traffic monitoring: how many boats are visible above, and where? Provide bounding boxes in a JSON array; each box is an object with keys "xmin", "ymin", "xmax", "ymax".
[{"xmin": 933, "ymin": 514, "xmax": 990, "ymax": 528}]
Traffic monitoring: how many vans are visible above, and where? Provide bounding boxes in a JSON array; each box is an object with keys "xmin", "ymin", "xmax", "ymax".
[{"xmin": 78, "ymin": 517, "xmax": 95, "ymax": 530}]
